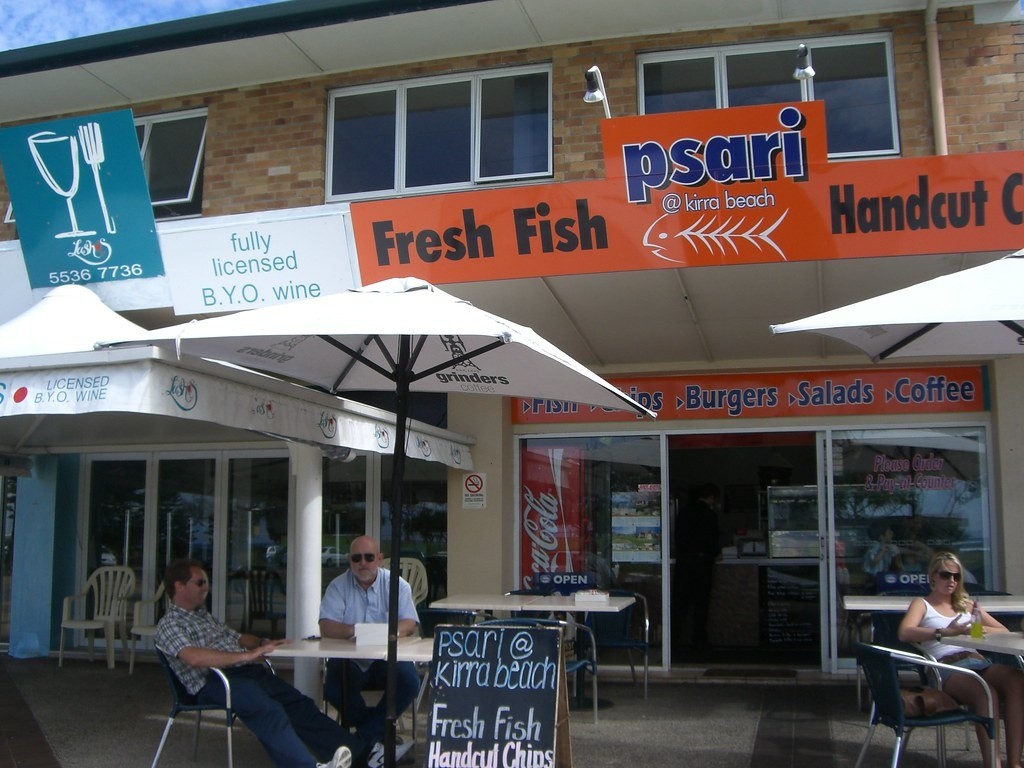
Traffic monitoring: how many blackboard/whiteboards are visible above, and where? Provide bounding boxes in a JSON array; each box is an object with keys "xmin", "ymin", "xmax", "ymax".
[
  {"xmin": 428, "ymin": 624, "xmax": 574, "ymax": 768},
  {"xmin": 756, "ymin": 564, "xmax": 821, "ymax": 665}
]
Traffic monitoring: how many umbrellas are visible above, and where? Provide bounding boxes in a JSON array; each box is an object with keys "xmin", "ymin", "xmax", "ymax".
[
  {"xmin": 835, "ymin": 428, "xmax": 989, "ymax": 536},
  {"xmin": 769, "ymin": 248, "xmax": 1023, "ymax": 364},
  {"xmin": 92, "ymin": 276, "xmax": 657, "ymax": 768},
  {"xmin": 120, "ymin": 467, "xmax": 249, "ymax": 556}
]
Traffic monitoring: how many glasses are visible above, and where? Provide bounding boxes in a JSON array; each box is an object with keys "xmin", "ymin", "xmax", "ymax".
[
  {"xmin": 936, "ymin": 571, "xmax": 961, "ymax": 581},
  {"xmin": 195, "ymin": 579, "xmax": 206, "ymax": 586},
  {"xmin": 351, "ymin": 553, "xmax": 375, "ymax": 563}
]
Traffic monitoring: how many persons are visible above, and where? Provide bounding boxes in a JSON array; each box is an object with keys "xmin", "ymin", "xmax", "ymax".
[
  {"xmin": 836, "ymin": 523, "xmax": 979, "ymax": 598},
  {"xmin": 319, "ymin": 536, "xmax": 420, "ymax": 768},
  {"xmin": 580, "ymin": 519, "xmax": 621, "ymax": 662},
  {"xmin": 669, "ymin": 483, "xmax": 723, "ymax": 665},
  {"xmin": 898, "ymin": 552, "xmax": 1024, "ymax": 768},
  {"xmin": 153, "ymin": 556, "xmax": 418, "ymax": 768}
]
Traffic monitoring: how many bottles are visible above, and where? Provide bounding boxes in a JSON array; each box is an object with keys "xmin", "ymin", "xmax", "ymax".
[{"xmin": 971, "ymin": 598, "xmax": 983, "ymax": 638}]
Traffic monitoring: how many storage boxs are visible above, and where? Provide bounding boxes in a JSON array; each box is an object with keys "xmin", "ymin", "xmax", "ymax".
[
  {"xmin": 611, "ymin": 493, "xmax": 661, "ymax": 516},
  {"xmin": 638, "ymin": 484, "xmax": 661, "ymax": 493},
  {"xmin": 611, "ymin": 517, "xmax": 662, "ymax": 562}
]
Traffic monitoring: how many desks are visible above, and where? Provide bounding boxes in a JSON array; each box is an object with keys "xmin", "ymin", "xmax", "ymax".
[
  {"xmin": 263, "ymin": 636, "xmax": 434, "ymax": 768},
  {"xmin": 942, "ymin": 632, "xmax": 1024, "ymax": 656},
  {"xmin": 431, "ymin": 594, "xmax": 636, "ymax": 701},
  {"xmin": 844, "ymin": 595, "xmax": 1024, "ymax": 710}
]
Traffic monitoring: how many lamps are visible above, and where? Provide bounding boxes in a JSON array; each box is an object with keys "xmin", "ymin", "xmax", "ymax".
[
  {"xmin": 582, "ymin": 65, "xmax": 611, "ymax": 118},
  {"xmin": 793, "ymin": 43, "xmax": 815, "ymax": 102}
]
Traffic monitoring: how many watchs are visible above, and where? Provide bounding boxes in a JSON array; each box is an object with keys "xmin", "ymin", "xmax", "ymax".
[{"xmin": 935, "ymin": 628, "xmax": 942, "ymax": 642}]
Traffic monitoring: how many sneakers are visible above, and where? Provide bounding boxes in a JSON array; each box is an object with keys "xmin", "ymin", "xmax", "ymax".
[
  {"xmin": 367, "ymin": 740, "xmax": 415, "ymax": 768},
  {"xmin": 316, "ymin": 746, "xmax": 352, "ymax": 768}
]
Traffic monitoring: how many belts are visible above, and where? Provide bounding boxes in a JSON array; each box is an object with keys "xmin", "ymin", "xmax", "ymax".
[{"xmin": 927, "ymin": 650, "xmax": 985, "ymax": 674}]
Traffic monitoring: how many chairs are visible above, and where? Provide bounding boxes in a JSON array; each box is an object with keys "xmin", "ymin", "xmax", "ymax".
[
  {"xmin": 380, "ymin": 557, "xmax": 428, "ymax": 606},
  {"xmin": 910, "ymin": 642, "xmax": 971, "ymax": 751},
  {"xmin": 148, "ymin": 636, "xmax": 277, "ymax": 768},
  {"xmin": 472, "ymin": 588, "xmax": 653, "ymax": 721},
  {"xmin": 128, "ymin": 581, "xmax": 166, "ymax": 675},
  {"xmin": 236, "ymin": 566, "xmax": 287, "ymax": 641},
  {"xmin": 852, "ymin": 643, "xmax": 996, "ymax": 768},
  {"xmin": 59, "ymin": 567, "xmax": 136, "ymax": 669}
]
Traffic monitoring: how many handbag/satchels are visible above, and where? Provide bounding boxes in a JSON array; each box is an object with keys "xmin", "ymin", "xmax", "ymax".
[{"xmin": 900, "ymin": 683, "xmax": 965, "ymax": 719}]
